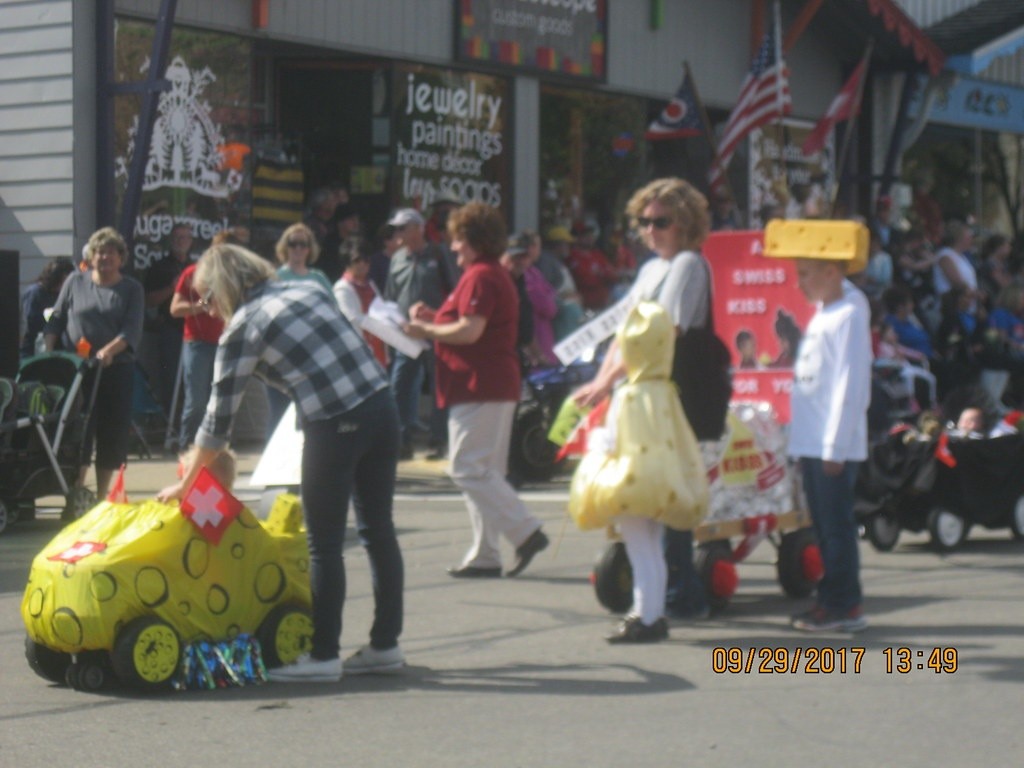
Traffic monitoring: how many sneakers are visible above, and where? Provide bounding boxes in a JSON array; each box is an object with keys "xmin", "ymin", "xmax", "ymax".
[
  {"xmin": 264, "ymin": 658, "xmax": 342, "ymax": 682},
  {"xmin": 342, "ymin": 644, "xmax": 404, "ymax": 674},
  {"xmin": 790, "ymin": 605, "xmax": 867, "ymax": 631}
]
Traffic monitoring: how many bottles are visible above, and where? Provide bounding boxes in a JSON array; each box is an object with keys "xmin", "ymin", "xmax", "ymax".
[
  {"xmin": 77, "ymin": 337, "xmax": 91, "ymax": 358},
  {"xmin": 34, "ymin": 332, "xmax": 45, "ymax": 353}
]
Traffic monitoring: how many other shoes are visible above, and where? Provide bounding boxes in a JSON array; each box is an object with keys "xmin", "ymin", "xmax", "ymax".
[
  {"xmin": 446, "ymin": 566, "xmax": 503, "ymax": 578},
  {"xmin": 605, "ymin": 616, "xmax": 667, "ymax": 643},
  {"xmin": 504, "ymin": 531, "xmax": 548, "ymax": 577}
]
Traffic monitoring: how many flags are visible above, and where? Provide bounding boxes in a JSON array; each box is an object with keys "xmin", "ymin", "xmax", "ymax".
[
  {"xmin": 804, "ymin": 63, "xmax": 866, "ymax": 155},
  {"xmin": 651, "ymin": 23, "xmax": 790, "ymax": 188}
]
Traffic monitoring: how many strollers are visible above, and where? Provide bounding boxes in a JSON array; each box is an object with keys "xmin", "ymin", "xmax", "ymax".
[{"xmin": 1, "ymin": 351, "xmax": 102, "ymax": 531}]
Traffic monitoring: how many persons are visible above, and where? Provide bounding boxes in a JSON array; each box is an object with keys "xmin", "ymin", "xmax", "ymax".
[{"xmin": 19, "ymin": 172, "xmax": 1024, "ymax": 681}]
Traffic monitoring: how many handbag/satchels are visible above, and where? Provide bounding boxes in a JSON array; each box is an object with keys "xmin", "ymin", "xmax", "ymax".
[{"xmin": 652, "ymin": 253, "xmax": 735, "ymax": 443}]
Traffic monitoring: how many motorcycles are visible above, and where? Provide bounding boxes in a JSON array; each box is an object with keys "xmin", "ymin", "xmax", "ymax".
[{"xmin": 508, "ymin": 358, "xmax": 604, "ymax": 483}]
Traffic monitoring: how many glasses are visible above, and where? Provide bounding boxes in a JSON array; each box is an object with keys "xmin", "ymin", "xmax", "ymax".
[
  {"xmin": 637, "ymin": 216, "xmax": 674, "ymax": 230},
  {"xmin": 288, "ymin": 241, "xmax": 309, "ymax": 248}
]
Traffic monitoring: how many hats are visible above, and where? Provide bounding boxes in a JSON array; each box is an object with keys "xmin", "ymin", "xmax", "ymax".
[
  {"xmin": 507, "ymin": 234, "xmax": 530, "ymax": 256},
  {"xmin": 388, "ymin": 209, "xmax": 423, "ymax": 226},
  {"xmin": 549, "ymin": 228, "xmax": 575, "ymax": 242}
]
